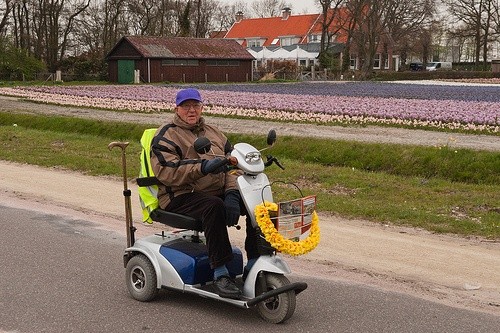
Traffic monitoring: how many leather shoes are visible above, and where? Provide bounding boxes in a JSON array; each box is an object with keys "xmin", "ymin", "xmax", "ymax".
[{"xmin": 212, "ymin": 274, "xmax": 242, "ymax": 299}]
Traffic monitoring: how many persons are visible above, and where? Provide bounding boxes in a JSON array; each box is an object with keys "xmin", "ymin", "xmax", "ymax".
[{"xmin": 150, "ymin": 89, "xmax": 242, "ymax": 297}]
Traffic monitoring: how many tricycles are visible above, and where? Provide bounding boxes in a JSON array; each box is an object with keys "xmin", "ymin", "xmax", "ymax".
[{"xmin": 123, "ymin": 129, "xmax": 308, "ymax": 324}]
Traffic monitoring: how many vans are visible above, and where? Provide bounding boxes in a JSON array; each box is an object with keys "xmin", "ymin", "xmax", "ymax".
[
  {"xmin": 410, "ymin": 62, "xmax": 423, "ymax": 72},
  {"xmin": 426, "ymin": 61, "xmax": 452, "ymax": 72}
]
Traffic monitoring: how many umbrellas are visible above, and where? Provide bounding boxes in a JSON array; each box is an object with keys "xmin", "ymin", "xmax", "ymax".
[{"xmin": 246, "ymin": 45, "xmax": 314, "ymax": 69}]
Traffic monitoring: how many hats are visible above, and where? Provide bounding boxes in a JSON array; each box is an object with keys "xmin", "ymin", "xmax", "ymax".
[{"xmin": 177, "ymin": 89, "xmax": 202, "ymax": 106}]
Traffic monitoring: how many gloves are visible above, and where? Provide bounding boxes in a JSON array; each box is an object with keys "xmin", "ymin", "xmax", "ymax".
[
  {"xmin": 202, "ymin": 157, "xmax": 228, "ymax": 176},
  {"xmin": 224, "ymin": 188, "xmax": 242, "ymax": 227}
]
